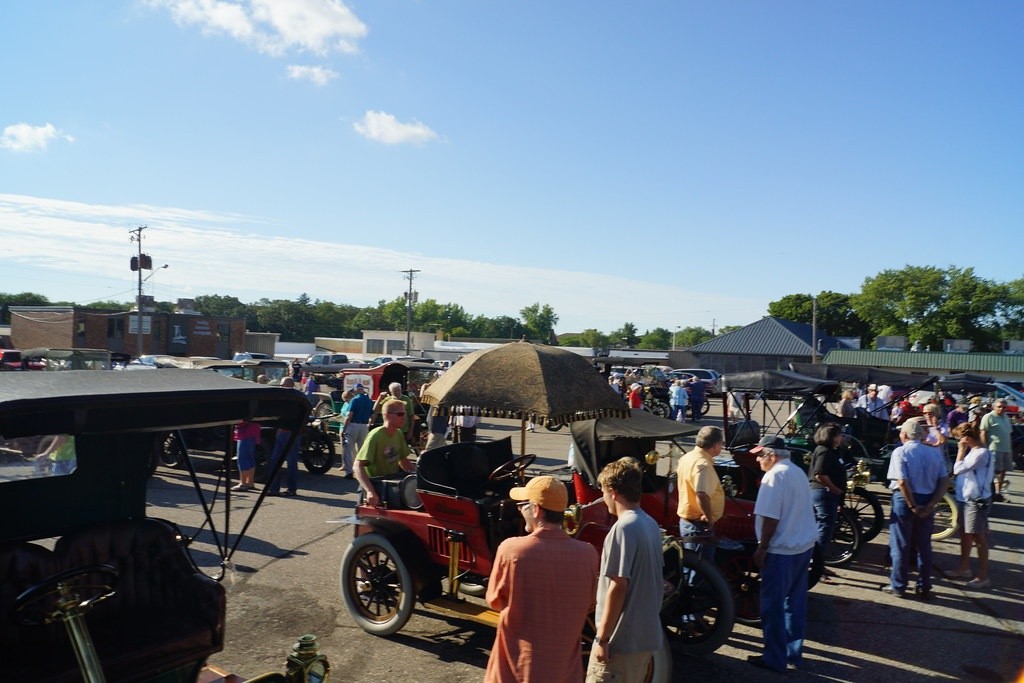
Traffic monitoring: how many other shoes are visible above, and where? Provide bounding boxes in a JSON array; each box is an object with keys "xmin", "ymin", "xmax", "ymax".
[
  {"xmin": 247, "ymin": 482, "xmax": 255, "ymax": 489},
  {"xmin": 231, "ymin": 483, "xmax": 248, "ymax": 492},
  {"xmin": 966, "ymin": 577, "xmax": 991, "ymax": 588},
  {"xmin": 748, "ymin": 654, "xmax": 786, "ymax": 674},
  {"xmin": 282, "ymin": 488, "xmax": 296, "ymax": 496},
  {"xmin": 260, "ymin": 486, "xmax": 279, "ymax": 496},
  {"xmin": 944, "ymin": 568, "xmax": 972, "ymax": 579}
]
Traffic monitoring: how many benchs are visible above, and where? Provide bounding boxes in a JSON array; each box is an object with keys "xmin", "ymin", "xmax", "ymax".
[
  {"xmin": 727, "ymin": 421, "xmax": 760, "ymax": 468},
  {"xmin": 418, "ymin": 442, "xmax": 516, "ymax": 528},
  {"xmin": 0, "ymin": 523, "xmax": 225, "ymax": 683},
  {"xmin": 796, "ymin": 407, "xmax": 818, "ymax": 439}
]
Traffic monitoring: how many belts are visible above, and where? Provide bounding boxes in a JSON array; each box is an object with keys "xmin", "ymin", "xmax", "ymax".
[{"xmin": 680, "ymin": 517, "xmax": 708, "ymax": 523}]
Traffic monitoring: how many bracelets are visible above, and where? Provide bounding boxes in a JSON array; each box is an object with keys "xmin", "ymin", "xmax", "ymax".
[
  {"xmin": 909, "ymin": 504, "xmax": 917, "ymax": 509},
  {"xmin": 594, "ymin": 636, "xmax": 610, "ymax": 644},
  {"xmin": 756, "ymin": 544, "xmax": 769, "ymax": 550}
]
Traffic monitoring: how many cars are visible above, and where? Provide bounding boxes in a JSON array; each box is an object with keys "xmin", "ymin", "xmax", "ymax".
[
  {"xmin": 0, "ymin": 336, "xmax": 1024, "ymax": 666},
  {"xmin": 1, "ymin": 365, "xmax": 315, "ymax": 683}
]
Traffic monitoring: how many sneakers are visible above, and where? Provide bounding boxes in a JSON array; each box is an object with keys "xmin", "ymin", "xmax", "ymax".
[{"xmin": 994, "ymin": 493, "xmax": 1010, "ymax": 503}]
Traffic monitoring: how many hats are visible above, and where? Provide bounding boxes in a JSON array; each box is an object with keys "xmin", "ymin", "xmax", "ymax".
[
  {"xmin": 867, "ymin": 384, "xmax": 882, "ymax": 393},
  {"xmin": 348, "ymin": 383, "xmax": 363, "ymax": 393},
  {"xmin": 509, "ymin": 476, "xmax": 568, "ymax": 512},
  {"xmin": 630, "ymin": 383, "xmax": 642, "ymax": 391},
  {"xmin": 749, "ymin": 436, "xmax": 786, "ymax": 454}
]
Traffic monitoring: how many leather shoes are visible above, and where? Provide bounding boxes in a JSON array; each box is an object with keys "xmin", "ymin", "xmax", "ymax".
[
  {"xmin": 879, "ymin": 584, "xmax": 904, "ymax": 598},
  {"xmin": 916, "ymin": 586, "xmax": 930, "ymax": 601}
]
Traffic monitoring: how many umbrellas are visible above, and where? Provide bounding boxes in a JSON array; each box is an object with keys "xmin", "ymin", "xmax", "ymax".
[{"xmin": 420, "ymin": 337, "xmax": 631, "ymax": 487}]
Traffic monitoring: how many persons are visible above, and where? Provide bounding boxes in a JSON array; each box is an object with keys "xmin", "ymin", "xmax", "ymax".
[
  {"xmin": 676, "ymin": 426, "xmax": 725, "ymax": 625},
  {"xmin": 728, "ymin": 392, "xmax": 742, "ymax": 421},
  {"xmin": 231, "ymin": 358, "xmax": 320, "ymax": 496},
  {"xmin": 609, "ymin": 369, "xmax": 705, "ymax": 422},
  {"xmin": 35, "ymin": 433, "xmax": 76, "ymax": 475},
  {"xmin": 525, "ymin": 415, "xmax": 535, "ymax": 432},
  {"xmin": 433, "ymin": 364, "xmax": 445, "ymax": 377},
  {"xmin": 749, "ymin": 436, "xmax": 818, "ymax": 669},
  {"xmin": 839, "ymin": 382, "xmax": 1012, "ymax": 598},
  {"xmin": 484, "ymin": 457, "xmax": 665, "ymax": 683},
  {"xmin": 328, "ymin": 384, "xmax": 373, "ymax": 478},
  {"xmin": 371, "ymin": 382, "xmax": 414, "ymax": 441},
  {"xmin": 419, "ymin": 372, "xmax": 450, "ymax": 451},
  {"xmin": 809, "ymin": 424, "xmax": 846, "ymax": 577},
  {"xmin": 456, "ymin": 405, "xmax": 480, "ymax": 442},
  {"xmin": 352, "ymin": 400, "xmax": 416, "ymax": 599}
]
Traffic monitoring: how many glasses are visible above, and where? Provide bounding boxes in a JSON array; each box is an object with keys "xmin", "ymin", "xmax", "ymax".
[
  {"xmin": 923, "ymin": 411, "xmax": 933, "ymax": 415},
  {"xmin": 996, "ymin": 406, "xmax": 1006, "ymax": 409},
  {"xmin": 387, "ymin": 411, "xmax": 405, "ymax": 417},
  {"xmin": 517, "ymin": 501, "xmax": 531, "ymax": 511}
]
